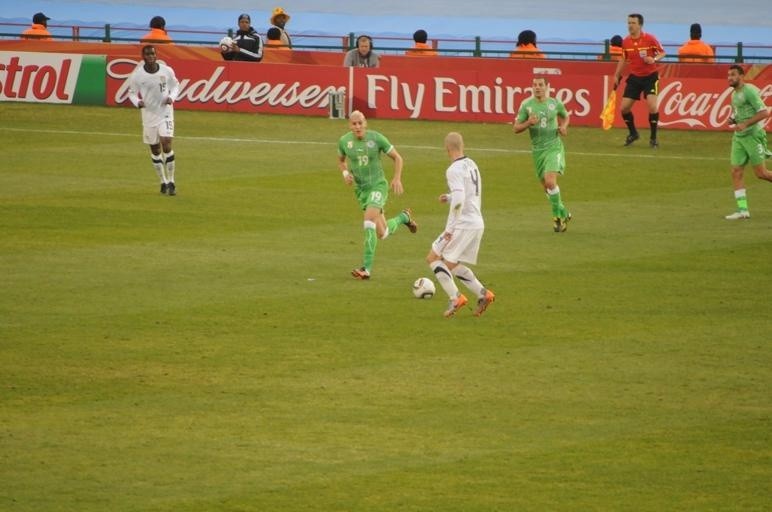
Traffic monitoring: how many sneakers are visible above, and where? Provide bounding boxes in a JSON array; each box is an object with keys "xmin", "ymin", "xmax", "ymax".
[
  {"xmin": 624, "ymin": 133, "xmax": 640, "ymax": 146},
  {"xmin": 553, "ymin": 210, "xmax": 572, "ymax": 232},
  {"xmin": 649, "ymin": 138, "xmax": 659, "ymax": 150},
  {"xmin": 725, "ymin": 209, "xmax": 751, "ymax": 219},
  {"xmin": 444, "ymin": 295, "xmax": 468, "ymax": 319},
  {"xmin": 404, "ymin": 208, "xmax": 417, "ymax": 233},
  {"xmin": 472, "ymin": 290, "xmax": 495, "ymax": 317},
  {"xmin": 160, "ymin": 182, "xmax": 176, "ymax": 196},
  {"xmin": 351, "ymin": 266, "xmax": 370, "ymax": 280}
]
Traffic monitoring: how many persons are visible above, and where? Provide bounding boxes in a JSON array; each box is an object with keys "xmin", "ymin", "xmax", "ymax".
[
  {"xmin": 219, "ymin": 13, "xmax": 263, "ymax": 61},
  {"xmin": 336, "ymin": 109, "xmax": 417, "ymax": 279},
  {"xmin": 726, "ymin": 65, "xmax": 772, "ymax": 221},
  {"xmin": 612, "ymin": 13, "xmax": 665, "ymax": 146},
  {"xmin": 141, "ymin": 16, "xmax": 173, "ymax": 44},
  {"xmin": 678, "ymin": 23, "xmax": 714, "ymax": 63},
  {"xmin": 405, "ymin": 29, "xmax": 436, "ymax": 57},
  {"xmin": 597, "ymin": 35, "xmax": 624, "ymax": 60},
  {"xmin": 426, "ymin": 133, "xmax": 495, "ymax": 317},
  {"xmin": 511, "ymin": 30, "xmax": 544, "ymax": 58},
  {"xmin": 513, "ymin": 76, "xmax": 572, "ymax": 232},
  {"xmin": 344, "ymin": 35, "xmax": 377, "ymax": 68},
  {"xmin": 128, "ymin": 46, "xmax": 180, "ymax": 195},
  {"xmin": 267, "ymin": 7, "xmax": 291, "ymax": 49},
  {"xmin": 21, "ymin": 12, "xmax": 52, "ymax": 41}
]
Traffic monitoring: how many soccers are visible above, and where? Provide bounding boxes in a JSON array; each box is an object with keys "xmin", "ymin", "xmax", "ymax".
[
  {"xmin": 413, "ymin": 277, "xmax": 435, "ymax": 298},
  {"xmin": 219, "ymin": 37, "xmax": 235, "ymax": 53}
]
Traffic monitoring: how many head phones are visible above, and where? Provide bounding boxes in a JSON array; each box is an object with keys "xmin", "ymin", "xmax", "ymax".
[{"xmin": 356, "ymin": 35, "xmax": 373, "ymax": 50}]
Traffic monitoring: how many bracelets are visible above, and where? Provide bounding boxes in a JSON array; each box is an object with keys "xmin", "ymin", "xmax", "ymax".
[
  {"xmin": 342, "ymin": 170, "xmax": 349, "ymax": 176},
  {"xmin": 654, "ymin": 57, "xmax": 656, "ymax": 61}
]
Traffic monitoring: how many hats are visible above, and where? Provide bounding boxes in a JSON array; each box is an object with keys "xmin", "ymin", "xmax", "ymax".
[
  {"xmin": 238, "ymin": 13, "xmax": 251, "ymax": 24},
  {"xmin": 270, "ymin": 7, "xmax": 291, "ymax": 25}
]
